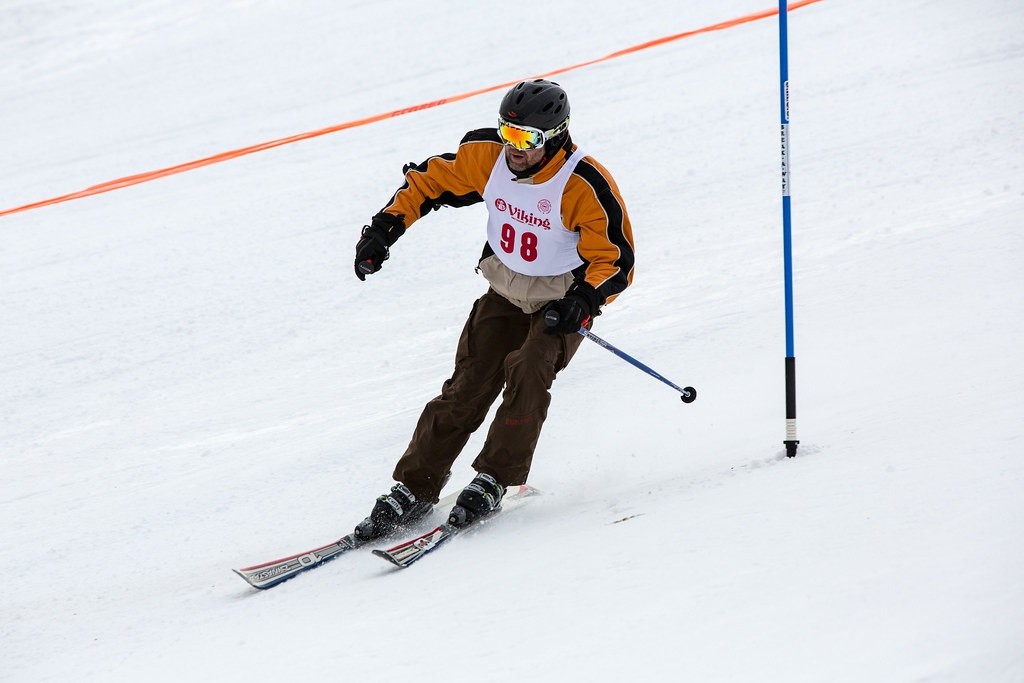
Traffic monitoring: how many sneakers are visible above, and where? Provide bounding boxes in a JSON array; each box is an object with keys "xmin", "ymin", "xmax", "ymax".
[
  {"xmin": 355, "ymin": 483, "xmax": 434, "ymax": 540},
  {"xmin": 448, "ymin": 473, "xmax": 506, "ymax": 530}
]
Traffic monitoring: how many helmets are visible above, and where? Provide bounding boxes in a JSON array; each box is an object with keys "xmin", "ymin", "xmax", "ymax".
[{"xmin": 498, "ymin": 78, "xmax": 570, "ymax": 129}]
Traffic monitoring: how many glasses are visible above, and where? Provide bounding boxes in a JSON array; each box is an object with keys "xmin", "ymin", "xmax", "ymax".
[{"xmin": 498, "ymin": 117, "xmax": 569, "ymax": 150}]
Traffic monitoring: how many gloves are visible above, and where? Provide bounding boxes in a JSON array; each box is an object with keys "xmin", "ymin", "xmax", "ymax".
[
  {"xmin": 355, "ymin": 213, "xmax": 406, "ymax": 281},
  {"xmin": 542, "ymin": 277, "xmax": 606, "ymax": 334}
]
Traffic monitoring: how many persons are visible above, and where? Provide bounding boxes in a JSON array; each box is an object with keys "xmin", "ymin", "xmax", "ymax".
[{"xmin": 355, "ymin": 76, "xmax": 636, "ymax": 523}]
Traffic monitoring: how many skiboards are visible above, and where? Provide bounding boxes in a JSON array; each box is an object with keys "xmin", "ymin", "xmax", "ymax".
[{"xmin": 232, "ymin": 483, "xmax": 531, "ymax": 592}]
